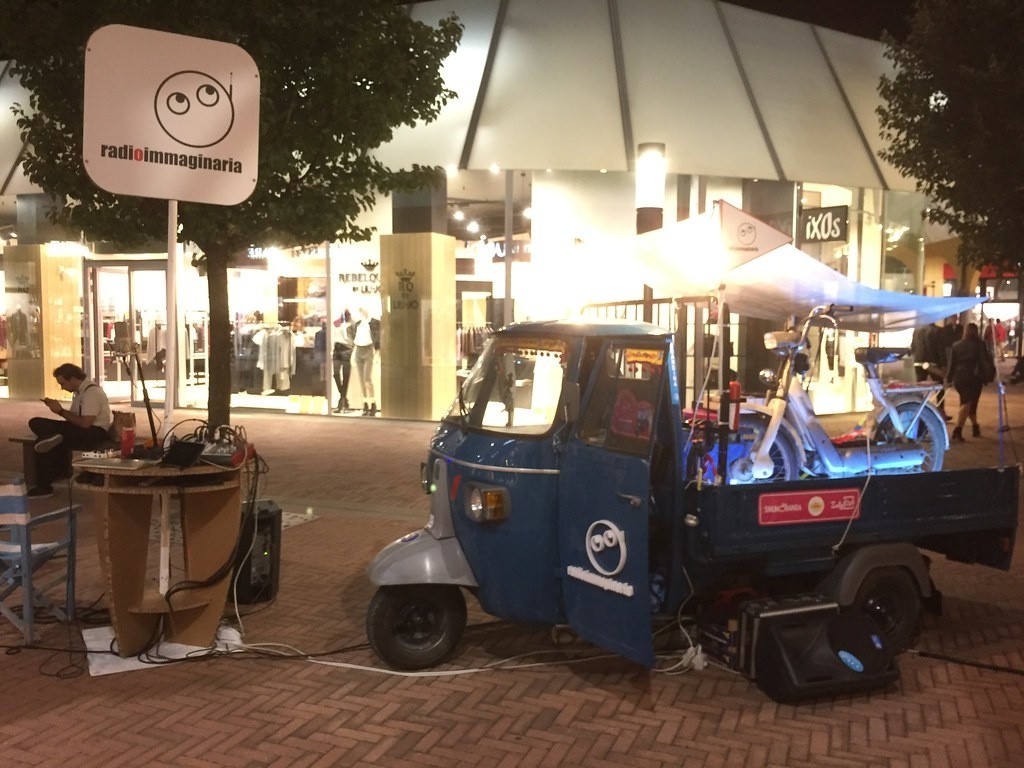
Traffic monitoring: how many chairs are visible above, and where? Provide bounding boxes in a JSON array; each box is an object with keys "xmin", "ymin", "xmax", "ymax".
[{"xmin": 0, "ymin": 478, "xmax": 81, "ymax": 648}]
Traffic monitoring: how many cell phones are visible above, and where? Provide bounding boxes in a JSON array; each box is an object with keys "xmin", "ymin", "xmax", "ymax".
[{"xmin": 40, "ymin": 399, "xmax": 48, "ymax": 403}]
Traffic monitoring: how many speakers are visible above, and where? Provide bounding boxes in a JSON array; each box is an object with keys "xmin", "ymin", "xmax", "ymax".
[
  {"xmin": 225, "ymin": 499, "xmax": 282, "ymax": 606},
  {"xmin": 757, "ymin": 612, "xmax": 902, "ymax": 704}
]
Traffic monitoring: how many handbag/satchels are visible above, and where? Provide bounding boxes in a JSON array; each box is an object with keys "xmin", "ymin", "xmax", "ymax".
[{"xmin": 972, "ymin": 341, "xmax": 992, "ymax": 388}]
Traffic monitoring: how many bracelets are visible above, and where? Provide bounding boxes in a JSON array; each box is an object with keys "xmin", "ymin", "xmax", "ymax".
[{"xmin": 58, "ymin": 409, "xmax": 64, "ymax": 416}]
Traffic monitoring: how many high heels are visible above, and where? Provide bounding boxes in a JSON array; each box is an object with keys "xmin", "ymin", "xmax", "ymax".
[
  {"xmin": 973, "ymin": 423, "xmax": 980, "ymax": 437},
  {"xmin": 952, "ymin": 426, "xmax": 964, "ymax": 441}
]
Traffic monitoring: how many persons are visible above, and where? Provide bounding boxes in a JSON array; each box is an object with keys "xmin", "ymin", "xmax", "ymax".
[
  {"xmin": 333, "ymin": 306, "xmax": 380, "ymax": 416},
  {"xmin": 27, "ymin": 364, "xmax": 110, "ymax": 499},
  {"xmin": 912, "ymin": 316, "xmax": 1006, "ymax": 441},
  {"xmin": 1010, "ymin": 355, "xmax": 1024, "ymax": 384}
]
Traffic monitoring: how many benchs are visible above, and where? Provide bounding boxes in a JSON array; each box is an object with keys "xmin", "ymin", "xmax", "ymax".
[{"xmin": 10, "ymin": 433, "xmax": 159, "ymax": 497}]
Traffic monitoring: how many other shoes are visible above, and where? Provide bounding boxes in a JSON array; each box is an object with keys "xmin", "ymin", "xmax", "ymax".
[
  {"xmin": 334, "ymin": 401, "xmax": 348, "ymax": 414},
  {"xmin": 944, "ymin": 416, "xmax": 952, "ymax": 420},
  {"xmin": 25, "ymin": 486, "xmax": 54, "ymax": 499},
  {"xmin": 369, "ymin": 402, "xmax": 376, "ymax": 415},
  {"xmin": 362, "ymin": 403, "xmax": 368, "ymax": 415},
  {"xmin": 34, "ymin": 434, "xmax": 63, "ymax": 454}
]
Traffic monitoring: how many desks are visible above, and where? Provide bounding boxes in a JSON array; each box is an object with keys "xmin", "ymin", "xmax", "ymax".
[{"xmin": 73, "ymin": 460, "xmax": 243, "ymax": 657}]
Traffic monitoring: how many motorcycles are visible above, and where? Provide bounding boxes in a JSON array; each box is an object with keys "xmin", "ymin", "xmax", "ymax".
[{"xmin": 364, "ymin": 320, "xmax": 1021, "ymax": 674}]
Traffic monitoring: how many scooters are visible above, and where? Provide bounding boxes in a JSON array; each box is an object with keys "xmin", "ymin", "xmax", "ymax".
[{"xmin": 712, "ymin": 303, "xmax": 949, "ymax": 485}]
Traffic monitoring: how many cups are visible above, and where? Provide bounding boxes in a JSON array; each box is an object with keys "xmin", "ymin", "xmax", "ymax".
[{"xmin": 120, "ymin": 430, "xmax": 134, "ymax": 460}]
